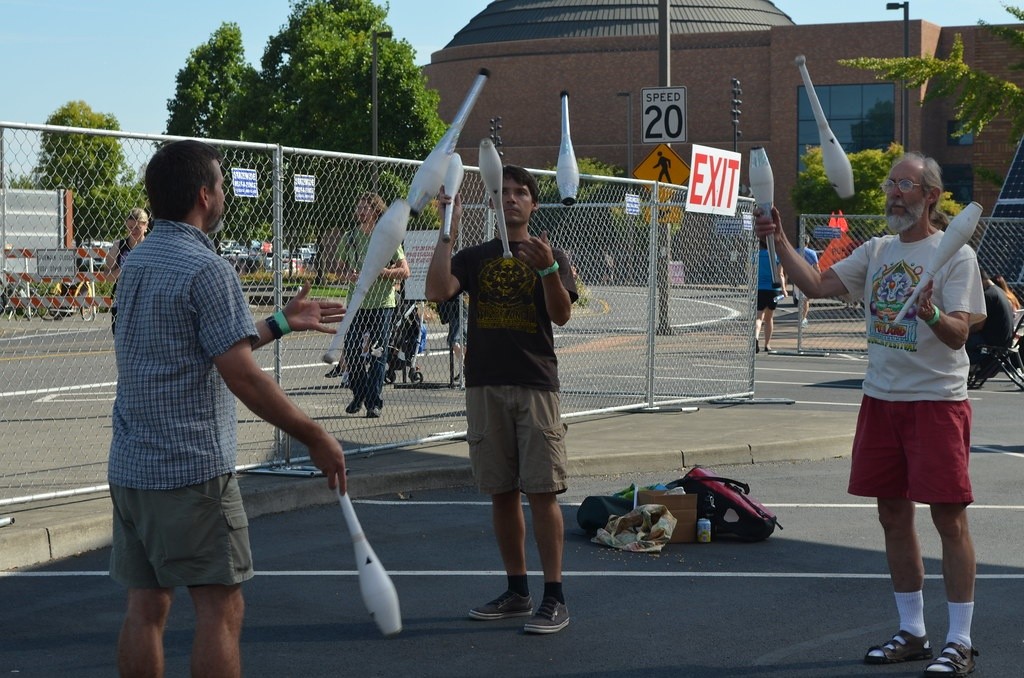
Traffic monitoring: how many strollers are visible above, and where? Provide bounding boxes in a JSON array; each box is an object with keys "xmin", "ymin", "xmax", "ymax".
[{"xmin": 340, "ymin": 300, "xmax": 427, "ymax": 388}]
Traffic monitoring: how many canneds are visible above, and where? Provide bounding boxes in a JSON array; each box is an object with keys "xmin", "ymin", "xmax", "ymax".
[{"xmin": 697, "ymin": 518, "xmax": 711, "ymax": 543}]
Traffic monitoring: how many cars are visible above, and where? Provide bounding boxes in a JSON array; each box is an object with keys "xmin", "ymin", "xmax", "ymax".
[
  {"xmin": 74, "ymin": 242, "xmax": 113, "ymax": 272},
  {"xmin": 220, "ymin": 239, "xmax": 317, "ymax": 273}
]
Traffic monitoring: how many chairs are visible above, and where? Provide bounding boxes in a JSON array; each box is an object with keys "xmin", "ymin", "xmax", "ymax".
[{"xmin": 966, "ymin": 309, "xmax": 1024, "ymax": 391}]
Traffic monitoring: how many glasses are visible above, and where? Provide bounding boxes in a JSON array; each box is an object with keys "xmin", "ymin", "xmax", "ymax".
[{"xmin": 881, "ymin": 179, "xmax": 922, "ymax": 193}]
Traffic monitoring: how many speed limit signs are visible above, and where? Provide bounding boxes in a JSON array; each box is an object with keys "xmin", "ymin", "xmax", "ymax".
[{"xmin": 641, "ymin": 86, "xmax": 689, "ymax": 145}]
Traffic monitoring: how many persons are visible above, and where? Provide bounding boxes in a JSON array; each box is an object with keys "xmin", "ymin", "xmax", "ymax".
[
  {"xmin": 785, "ymin": 235, "xmax": 822, "ymax": 325},
  {"xmin": 426, "ymin": 166, "xmax": 578, "ymax": 633},
  {"xmin": 337, "ymin": 194, "xmax": 410, "ymax": 416},
  {"xmin": 754, "ymin": 156, "xmax": 984, "ymax": 678},
  {"xmin": 751, "ymin": 238, "xmax": 789, "ymax": 354},
  {"xmin": 966, "ymin": 269, "xmax": 1020, "ymax": 372},
  {"xmin": 105, "ymin": 208, "xmax": 148, "ymax": 334},
  {"xmin": 603, "ymin": 250, "xmax": 614, "ymax": 284},
  {"xmin": 107, "ymin": 140, "xmax": 347, "ymax": 677}
]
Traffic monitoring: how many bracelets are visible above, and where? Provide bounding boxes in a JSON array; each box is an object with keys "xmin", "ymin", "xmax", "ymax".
[
  {"xmin": 925, "ymin": 305, "xmax": 939, "ymax": 324},
  {"xmin": 539, "ymin": 260, "xmax": 559, "ymax": 276},
  {"xmin": 266, "ymin": 311, "xmax": 291, "ymax": 339}
]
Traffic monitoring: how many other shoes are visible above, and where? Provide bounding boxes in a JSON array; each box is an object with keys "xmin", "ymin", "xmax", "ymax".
[
  {"xmin": 765, "ymin": 347, "xmax": 771, "ymax": 351},
  {"xmin": 326, "ymin": 365, "xmax": 343, "ymax": 378},
  {"xmin": 756, "ymin": 339, "xmax": 759, "ymax": 353},
  {"xmin": 345, "ymin": 399, "xmax": 362, "ymax": 414},
  {"xmin": 367, "ymin": 405, "xmax": 381, "ymax": 418},
  {"xmin": 802, "ymin": 319, "xmax": 808, "ymax": 328}
]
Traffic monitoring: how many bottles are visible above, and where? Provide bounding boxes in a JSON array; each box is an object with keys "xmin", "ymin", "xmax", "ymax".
[{"xmin": 773, "ymin": 294, "xmax": 785, "ymax": 303}]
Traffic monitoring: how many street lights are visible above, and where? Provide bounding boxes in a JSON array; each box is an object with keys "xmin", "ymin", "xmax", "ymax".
[
  {"xmin": 885, "ymin": 1, "xmax": 910, "ymax": 153},
  {"xmin": 732, "ymin": 78, "xmax": 744, "ymax": 151},
  {"xmin": 372, "ymin": 29, "xmax": 393, "ymax": 193}
]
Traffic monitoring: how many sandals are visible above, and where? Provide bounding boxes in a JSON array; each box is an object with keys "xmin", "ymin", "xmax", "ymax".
[
  {"xmin": 923, "ymin": 642, "xmax": 979, "ymax": 678},
  {"xmin": 865, "ymin": 629, "xmax": 933, "ymax": 664}
]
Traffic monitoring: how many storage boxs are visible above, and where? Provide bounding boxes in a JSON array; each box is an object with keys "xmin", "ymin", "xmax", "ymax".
[{"xmin": 638, "ymin": 488, "xmax": 698, "ymax": 543}]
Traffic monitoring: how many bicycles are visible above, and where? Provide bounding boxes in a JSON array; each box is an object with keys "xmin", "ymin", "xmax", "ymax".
[{"xmin": 37, "ymin": 281, "xmax": 92, "ymax": 322}]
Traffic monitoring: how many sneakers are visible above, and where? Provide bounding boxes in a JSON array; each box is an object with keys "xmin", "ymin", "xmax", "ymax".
[
  {"xmin": 524, "ymin": 597, "xmax": 569, "ymax": 634},
  {"xmin": 469, "ymin": 589, "xmax": 533, "ymax": 620}
]
